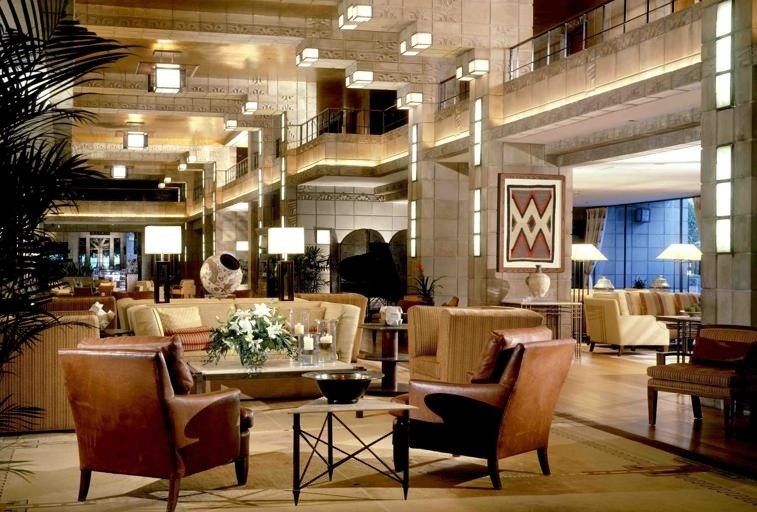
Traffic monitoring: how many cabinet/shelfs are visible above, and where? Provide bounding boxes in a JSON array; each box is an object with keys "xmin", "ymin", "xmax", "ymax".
[{"xmin": 360, "ymin": 321, "xmax": 410, "ymax": 397}]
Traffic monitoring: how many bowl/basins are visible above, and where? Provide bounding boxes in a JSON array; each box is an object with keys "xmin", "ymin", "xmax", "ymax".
[{"xmin": 302, "ymin": 370, "xmax": 386, "ymax": 404}]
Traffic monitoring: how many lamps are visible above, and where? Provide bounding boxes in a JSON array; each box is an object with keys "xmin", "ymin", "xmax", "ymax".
[
  {"xmin": 242, "ymin": 105, "xmax": 254, "ymax": 115},
  {"xmin": 123, "ymin": 130, "xmax": 148, "ymax": 149},
  {"xmin": 352, "ymin": 4, "xmax": 373, "ymax": 22},
  {"xmin": 396, "ymin": 97, "xmax": 410, "ymax": 110},
  {"xmin": 224, "ymin": 123, "xmax": 234, "ymax": 131},
  {"xmin": 571, "ymin": 243, "xmax": 608, "ymax": 343},
  {"xmin": 246, "ymin": 101, "xmax": 258, "ymax": 111},
  {"xmin": 636, "ymin": 208, "xmax": 650, "ymax": 223},
  {"xmin": 456, "ymin": 66, "xmax": 474, "ymax": 81},
  {"xmin": 227, "ymin": 119, "xmax": 237, "ymax": 129},
  {"xmin": 411, "ymin": 32, "xmax": 432, "ymax": 49},
  {"xmin": 268, "ymin": 226, "xmax": 304, "ymax": 302},
  {"xmin": 405, "ymin": 91, "xmax": 423, "ymax": 106},
  {"xmin": 352, "ymin": 71, "xmax": 374, "ymax": 83},
  {"xmin": 345, "ymin": 76, "xmax": 364, "ymax": 89},
  {"xmin": 144, "ymin": 225, "xmax": 182, "ymax": 304},
  {"xmin": 302, "ymin": 48, "xmax": 319, "ymax": 62},
  {"xmin": 147, "ymin": 63, "xmax": 187, "ymax": 94},
  {"xmin": 338, "ymin": 13, "xmax": 359, "ymax": 30},
  {"xmin": 400, "ymin": 40, "xmax": 419, "ymax": 56},
  {"xmin": 468, "ymin": 59, "xmax": 489, "ymax": 75},
  {"xmin": 347, "ymin": 5, "xmax": 353, "ymax": 21},
  {"xmin": 656, "ymin": 244, "xmax": 703, "ymax": 293},
  {"xmin": 296, "ymin": 52, "xmax": 313, "ymax": 68}
]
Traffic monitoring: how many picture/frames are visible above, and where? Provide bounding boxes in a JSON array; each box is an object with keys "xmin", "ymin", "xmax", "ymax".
[{"xmin": 498, "ymin": 172, "xmax": 566, "ymax": 274}]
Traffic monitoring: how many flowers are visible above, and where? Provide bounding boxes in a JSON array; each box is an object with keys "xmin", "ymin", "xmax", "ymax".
[
  {"xmin": 406, "ymin": 262, "xmax": 447, "ymax": 296},
  {"xmin": 202, "ymin": 303, "xmax": 300, "ymax": 358}
]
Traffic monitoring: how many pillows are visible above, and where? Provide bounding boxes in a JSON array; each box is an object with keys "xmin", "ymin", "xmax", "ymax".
[
  {"xmin": 300, "ymin": 308, "xmax": 326, "ymax": 332},
  {"xmin": 156, "ymin": 306, "xmax": 201, "ymax": 333},
  {"xmin": 165, "ymin": 327, "xmax": 213, "ymax": 353}
]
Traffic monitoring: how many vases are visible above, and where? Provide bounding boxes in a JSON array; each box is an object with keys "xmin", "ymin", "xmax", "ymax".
[
  {"xmin": 421, "ymin": 297, "xmax": 434, "ymax": 306},
  {"xmin": 252, "ymin": 351, "xmax": 268, "ymax": 366}
]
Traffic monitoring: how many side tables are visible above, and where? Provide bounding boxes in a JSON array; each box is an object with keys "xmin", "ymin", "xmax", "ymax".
[{"xmin": 288, "ymin": 394, "xmax": 419, "ymax": 506}]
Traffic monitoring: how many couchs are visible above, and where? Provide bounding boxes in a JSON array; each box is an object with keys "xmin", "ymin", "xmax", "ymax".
[
  {"xmin": 58, "ymin": 334, "xmax": 254, "ymax": 512},
  {"xmin": 42, "ymin": 295, "xmax": 117, "ymax": 330},
  {"xmin": 648, "ymin": 325, "xmax": 757, "ymax": 432},
  {"xmin": 1, "ymin": 309, "xmax": 100, "ymax": 434},
  {"xmin": 118, "ymin": 297, "xmax": 361, "ymax": 401},
  {"xmin": 389, "ymin": 327, "xmax": 578, "ymax": 491},
  {"xmin": 407, "ymin": 305, "xmax": 542, "ymax": 382},
  {"xmin": 625, "ymin": 292, "xmax": 701, "ymax": 352},
  {"xmin": 584, "ymin": 294, "xmax": 670, "ymax": 357}
]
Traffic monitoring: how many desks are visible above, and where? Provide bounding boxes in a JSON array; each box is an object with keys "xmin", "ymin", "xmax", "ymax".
[{"xmin": 501, "ymin": 298, "xmax": 583, "ymax": 360}]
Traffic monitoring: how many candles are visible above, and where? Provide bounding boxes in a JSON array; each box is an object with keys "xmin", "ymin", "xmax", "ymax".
[
  {"xmin": 302, "ymin": 335, "xmax": 315, "ymax": 351},
  {"xmin": 320, "ymin": 333, "xmax": 333, "ymax": 344},
  {"xmin": 295, "ymin": 323, "xmax": 305, "ymax": 335}
]
